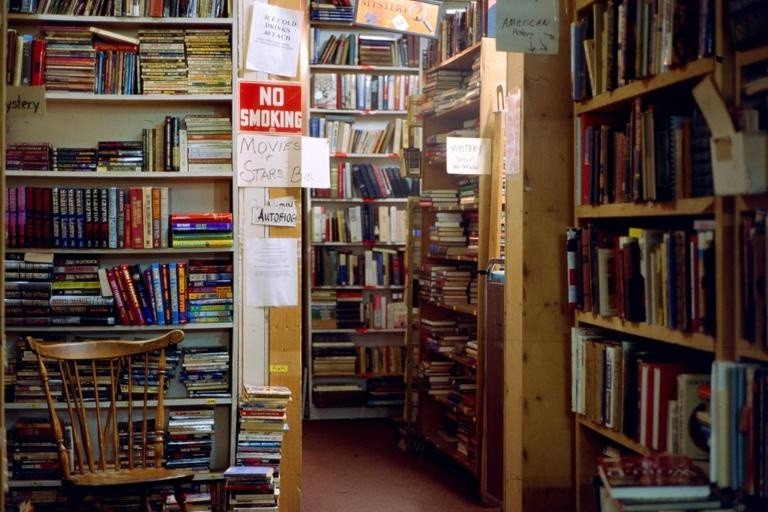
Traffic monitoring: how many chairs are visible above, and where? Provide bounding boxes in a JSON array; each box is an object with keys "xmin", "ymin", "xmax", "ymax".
[{"xmin": 18, "ymin": 331, "xmax": 196, "ymax": 510}]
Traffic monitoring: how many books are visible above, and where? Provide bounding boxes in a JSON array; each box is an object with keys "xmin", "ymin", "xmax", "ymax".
[
  {"xmin": 1, "ymin": 0, "xmax": 294, "ymax": 512},
  {"xmin": 563, "ymin": 0, "xmax": 768, "ymax": 512},
  {"xmin": 305, "ymin": 1, "xmax": 505, "ymax": 480}
]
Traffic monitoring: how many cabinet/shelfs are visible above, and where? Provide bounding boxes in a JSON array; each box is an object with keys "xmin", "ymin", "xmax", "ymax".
[
  {"xmin": 0, "ymin": 0, "xmax": 237, "ymax": 488},
  {"xmin": 567, "ymin": 3, "xmax": 725, "ymax": 510},
  {"xmin": 716, "ymin": 3, "xmax": 768, "ymax": 510},
  {"xmin": 406, "ymin": 42, "xmax": 504, "ymax": 505},
  {"xmin": 307, "ymin": 6, "xmax": 421, "ymax": 429}
]
